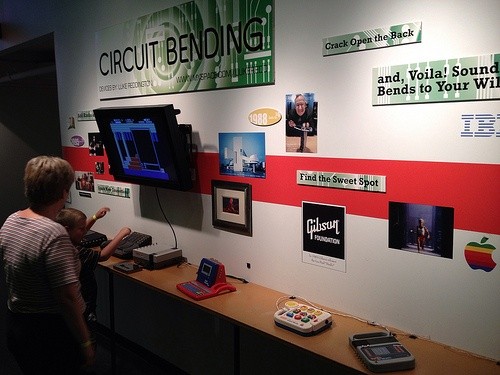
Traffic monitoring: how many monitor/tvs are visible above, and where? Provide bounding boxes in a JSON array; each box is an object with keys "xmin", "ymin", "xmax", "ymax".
[{"xmin": 93, "ymin": 104, "xmax": 193, "ymax": 192}]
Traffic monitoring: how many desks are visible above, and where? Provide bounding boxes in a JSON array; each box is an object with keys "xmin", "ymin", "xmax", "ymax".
[{"xmin": 93, "ymin": 240, "xmax": 500, "ymax": 375}]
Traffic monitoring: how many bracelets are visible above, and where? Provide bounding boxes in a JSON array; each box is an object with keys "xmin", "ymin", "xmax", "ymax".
[
  {"xmin": 81, "ymin": 340, "xmax": 91, "ymax": 348},
  {"xmin": 92, "ymin": 214, "xmax": 98, "ymax": 221}
]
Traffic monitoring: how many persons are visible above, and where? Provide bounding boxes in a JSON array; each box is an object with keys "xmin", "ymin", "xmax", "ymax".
[
  {"xmin": 55, "ymin": 208, "xmax": 132, "ymax": 375},
  {"xmin": 0, "ymin": 156, "xmax": 97, "ymax": 375},
  {"xmin": 288, "ymin": 94, "xmax": 315, "ymax": 135},
  {"xmin": 411, "ymin": 219, "xmax": 431, "ymax": 253}
]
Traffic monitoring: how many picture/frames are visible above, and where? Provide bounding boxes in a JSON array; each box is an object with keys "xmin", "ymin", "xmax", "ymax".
[{"xmin": 211, "ymin": 180, "xmax": 252, "ymax": 237}]
